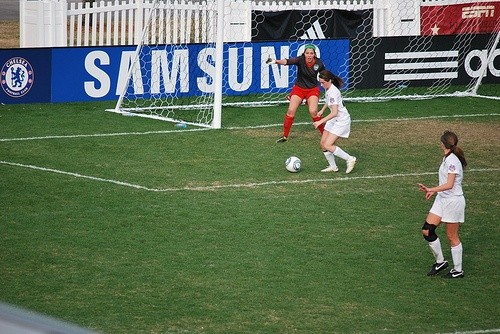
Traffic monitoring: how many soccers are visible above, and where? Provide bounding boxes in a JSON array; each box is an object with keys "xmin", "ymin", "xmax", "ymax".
[{"xmin": 285, "ymin": 156, "xmax": 302, "ymax": 173}]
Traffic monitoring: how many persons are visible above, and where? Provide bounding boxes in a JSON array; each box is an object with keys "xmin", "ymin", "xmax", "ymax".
[
  {"xmin": 417, "ymin": 130, "xmax": 465, "ymax": 278},
  {"xmin": 265, "ymin": 44, "xmax": 325, "ymax": 143},
  {"xmin": 312, "ymin": 69, "xmax": 357, "ymax": 174}
]
